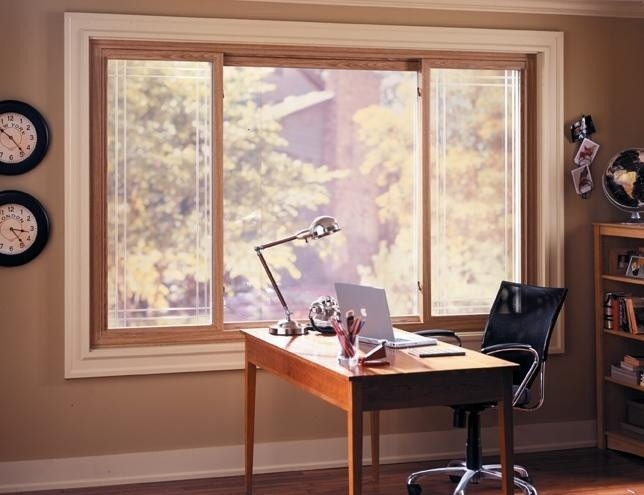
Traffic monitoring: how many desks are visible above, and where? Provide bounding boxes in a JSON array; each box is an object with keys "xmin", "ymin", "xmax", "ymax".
[{"xmin": 238, "ymin": 328, "xmax": 521, "ymax": 495}]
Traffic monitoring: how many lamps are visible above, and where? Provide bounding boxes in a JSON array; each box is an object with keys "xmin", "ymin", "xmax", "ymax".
[{"xmin": 254, "ymin": 216, "xmax": 341, "ymax": 335}]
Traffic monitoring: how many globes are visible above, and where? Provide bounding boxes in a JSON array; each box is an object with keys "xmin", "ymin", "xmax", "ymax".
[{"xmin": 602, "ymin": 148, "xmax": 644, "ymax": 224}]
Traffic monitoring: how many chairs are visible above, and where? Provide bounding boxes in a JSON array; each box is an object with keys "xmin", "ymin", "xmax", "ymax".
[{"xmin": 407, "ymin": 282, "xmax": 569, "ymax": 495}]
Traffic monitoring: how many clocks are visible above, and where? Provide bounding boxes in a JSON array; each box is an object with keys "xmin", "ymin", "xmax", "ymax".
[
  {"xmin": 0, "ymin": 189, "xmax": 51, "ymax": 268},
  {"xmin": 0, "ymin": 99, "xmax": 49, "ymax": 175}
]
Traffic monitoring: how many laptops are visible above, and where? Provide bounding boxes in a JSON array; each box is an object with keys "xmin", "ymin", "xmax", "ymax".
[{"xmin": 334, "ymin": 281, "xmax": 438, "ymax": 349}]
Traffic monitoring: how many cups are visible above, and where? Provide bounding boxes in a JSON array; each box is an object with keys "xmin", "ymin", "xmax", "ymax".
[{"xmin": 337, "ymin": 334, "xmax": 359, "ymax": 361}]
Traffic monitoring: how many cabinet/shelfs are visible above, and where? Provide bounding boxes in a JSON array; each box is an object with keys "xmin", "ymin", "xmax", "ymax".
[{"xmin": 591, "ymin": 222, "xmax": 644, "ymax": 460}]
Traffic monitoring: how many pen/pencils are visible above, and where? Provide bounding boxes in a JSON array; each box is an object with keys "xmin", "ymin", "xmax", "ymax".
[
  {"xmin": 419, "ymin": 352, "xmax": 465, "ymax": 358},
  {"xmin": 331, "ymin": 309, "xmax": 366, "ymax": 358}
]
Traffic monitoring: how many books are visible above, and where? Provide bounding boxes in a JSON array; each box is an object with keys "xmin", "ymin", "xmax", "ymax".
[
  {"xmin": 603, "ymin": 291, "xmax": 643, "ymax": 335},
  {"xmin": 610, "ymin": 351, "xmax": 644, "ymax": 388}
]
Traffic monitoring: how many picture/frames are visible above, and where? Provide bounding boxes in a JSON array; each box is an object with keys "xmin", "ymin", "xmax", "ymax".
[{"xmin": 625, "ymin": 255, "xmax": 644, "ymax": 279}]
